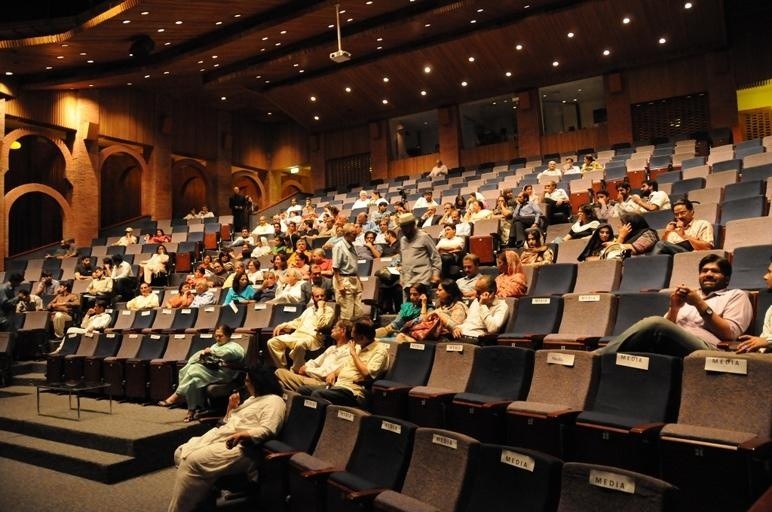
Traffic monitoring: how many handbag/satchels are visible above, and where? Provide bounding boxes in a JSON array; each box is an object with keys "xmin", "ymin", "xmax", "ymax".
[
  {"xmin": 399, "ymin": 312, "xmax": 442, "ymax": 341},
  {"xmin": 199, "ymin": 354, "xmax": 221, "ymax": 371}
]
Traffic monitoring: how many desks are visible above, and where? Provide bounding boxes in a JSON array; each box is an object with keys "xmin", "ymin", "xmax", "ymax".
[{"xmin": 35, "ymin": 379, "xmax": 113, "ymax": 421}]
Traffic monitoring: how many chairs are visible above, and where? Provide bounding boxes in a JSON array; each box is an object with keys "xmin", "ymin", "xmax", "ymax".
[
  {"xmin": 199, "ymin": 125, "xmax": 772, "ymax": 510},
  {"xmin": 3, "ymin": 215, "xmax": 199, "ymax": 407}
]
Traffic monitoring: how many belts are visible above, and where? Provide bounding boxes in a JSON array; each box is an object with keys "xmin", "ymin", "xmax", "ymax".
[{"xmin": 339, "ymin": 273, "xmax": 358, "ymax": 277}]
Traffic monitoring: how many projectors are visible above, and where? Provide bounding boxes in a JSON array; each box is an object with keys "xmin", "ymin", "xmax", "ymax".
[{"xmin": 330, "ymin": 51, "xmax": 351, "ymax": 63}]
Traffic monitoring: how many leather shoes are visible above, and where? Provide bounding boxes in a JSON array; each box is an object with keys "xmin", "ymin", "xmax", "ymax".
[
  {"xmin": 157, "ymin": 398, "xmax": 184, "ymax": 408},
  {"xmin": 184, "ymin": 408, "xmax": 197, "ymax": 423}
]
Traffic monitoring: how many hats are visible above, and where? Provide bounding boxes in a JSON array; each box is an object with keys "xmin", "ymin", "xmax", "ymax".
[{"xmin": 397, "ymin": 212, "xmax": 417, "ymax": 227}]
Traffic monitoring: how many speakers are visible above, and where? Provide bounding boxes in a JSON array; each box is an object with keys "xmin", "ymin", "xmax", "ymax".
[{"xmin": 84, "ymin": 123, "xmax": 100, "ymax": 141}]
